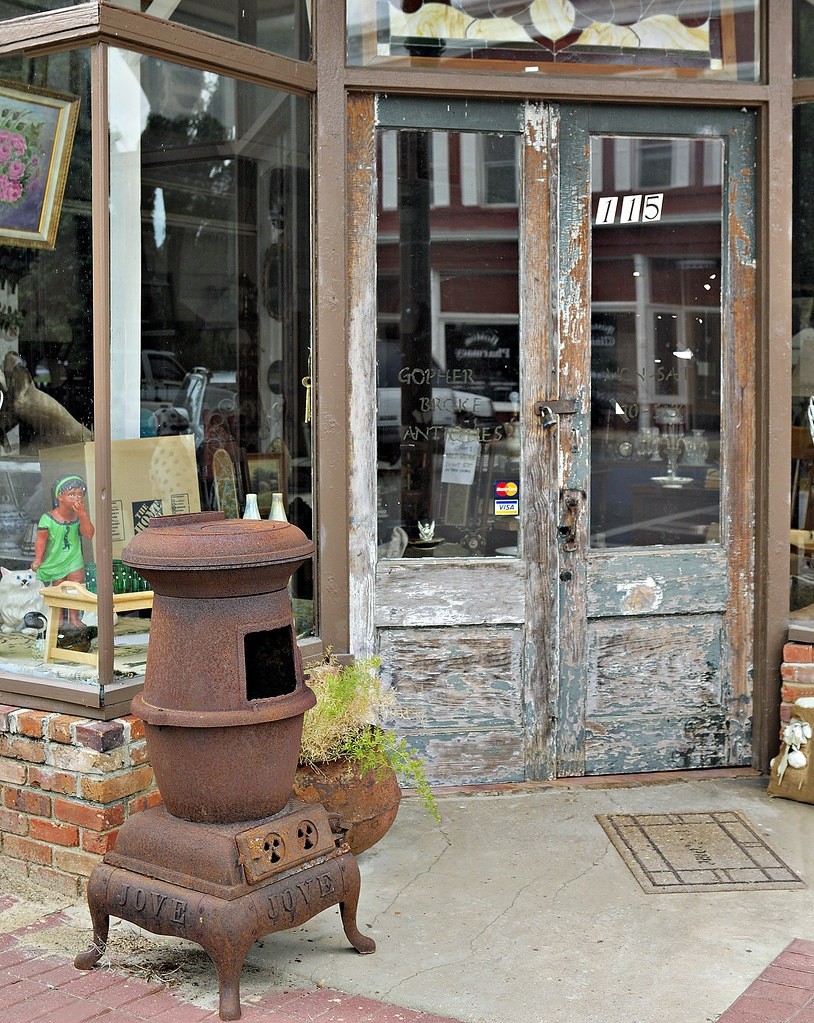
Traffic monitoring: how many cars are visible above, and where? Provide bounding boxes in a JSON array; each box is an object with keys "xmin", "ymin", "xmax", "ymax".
[{"xmin": 375, "ymin": 336, "xmax": 459, "ymax": 466}]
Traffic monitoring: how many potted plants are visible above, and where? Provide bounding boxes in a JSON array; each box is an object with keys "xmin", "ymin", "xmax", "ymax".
[{"xmin": 289, "ymin": 650, "xmax": 452, "ymax": 859}]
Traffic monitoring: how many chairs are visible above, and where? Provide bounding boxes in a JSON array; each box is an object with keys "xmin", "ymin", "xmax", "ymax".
[
  {"xmin": 195, "ymin": 404, "xmax": 247, "ymax": 521},
  {"xmin": 398, "ymin": 425, "xmax": 498, "ymax": 559}
]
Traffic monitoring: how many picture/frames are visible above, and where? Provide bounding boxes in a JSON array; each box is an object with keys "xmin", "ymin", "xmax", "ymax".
[
  {"xmin": 244, "ymin": 450, "xmax": 290, "ymax": 515},
  {"xmin": 0, "ymin": 74, "xmax": 84, "ymax": 252}
]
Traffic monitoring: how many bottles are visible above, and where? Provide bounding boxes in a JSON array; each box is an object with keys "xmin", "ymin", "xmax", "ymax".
[
  {"xmin": 242, "ymin": 493, "xmax": 262, "ymax": 521},
  {"xmin": 267, "ymin": 493, "xmax": 289, "ymax": 522}
]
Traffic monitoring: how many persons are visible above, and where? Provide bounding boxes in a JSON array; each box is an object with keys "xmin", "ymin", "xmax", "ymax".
[{"xmin": 30, "ymin": 474, "xmax": 96, "ymax": 632}]
